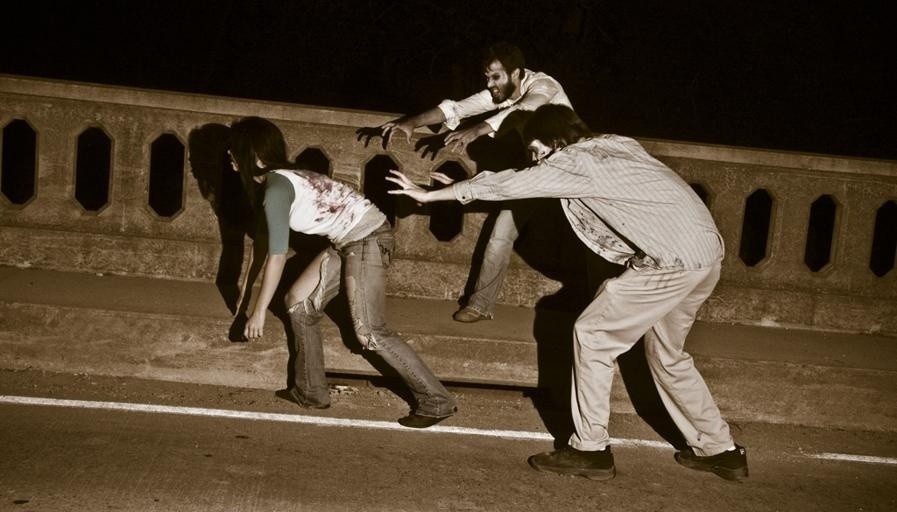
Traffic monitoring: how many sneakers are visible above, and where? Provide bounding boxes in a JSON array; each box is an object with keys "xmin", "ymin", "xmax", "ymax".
[
  {"xmin": 527, "ymin": 445, "xmax": 616, "ymax": 482},
  {"xmin": 674, "ymin": 443, "xmax": 749, "ymax": 482}
]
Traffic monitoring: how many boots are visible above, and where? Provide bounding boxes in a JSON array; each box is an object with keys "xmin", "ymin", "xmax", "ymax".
[{"xmin": 453, "ymin": 306, "xmax": 495, "ymax": 323}]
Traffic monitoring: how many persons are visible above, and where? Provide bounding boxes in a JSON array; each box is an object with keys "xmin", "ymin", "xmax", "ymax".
[
  {"xmin": 380, "ymin": 104, "xmax": 751, "ymax": 485},
  {"xmin": 378, "ymin": 40, "xmax": 576, "ymax": 323},
  {"xmin": 221, "ymin": 115, "xmax": 458, "ymax": 430}
]
